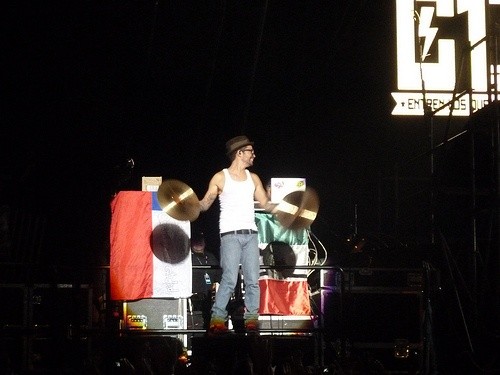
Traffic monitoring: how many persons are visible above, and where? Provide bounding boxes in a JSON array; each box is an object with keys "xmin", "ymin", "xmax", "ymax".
[
  {"xmin": 194, "ymin": 136, "xmax": 282, "ymax": 333},
  {"xmin": 190, "ymin": 230, "xmax": 223, "ymax": 311}
]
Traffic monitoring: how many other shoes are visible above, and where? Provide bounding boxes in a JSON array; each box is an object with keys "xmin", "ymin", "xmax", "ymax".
[
  {"xmin": 245, "ymin": 318, "xmax": 259, "ymax": 332},
  {"xmin": 210, "ymin": 318, "xmax": 229, "ymax": 333}
]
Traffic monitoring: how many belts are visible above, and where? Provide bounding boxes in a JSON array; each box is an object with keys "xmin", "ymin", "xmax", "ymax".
[{"xmin": 221, "ymin": 228, "xmax": 258, "ymax": 239}]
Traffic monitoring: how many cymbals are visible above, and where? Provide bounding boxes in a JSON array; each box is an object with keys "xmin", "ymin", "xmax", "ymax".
[
  {"xmin": 157, "ymin": 179, "xmax": 201, "ymax": 222},
  {"xmin": 277, "ymin": 191, "xmax": 319, "ymax": 230}
]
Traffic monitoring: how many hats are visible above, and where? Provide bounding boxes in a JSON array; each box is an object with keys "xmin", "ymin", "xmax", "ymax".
[{"xmin": 226, "ymin": 135, "xmax": 253, "ymax": 155}]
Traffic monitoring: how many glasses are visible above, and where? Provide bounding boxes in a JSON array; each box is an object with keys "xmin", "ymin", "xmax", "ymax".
[{"xmin": 240, "ymin": 149, "xmax": 254, "ymax": 154}]
